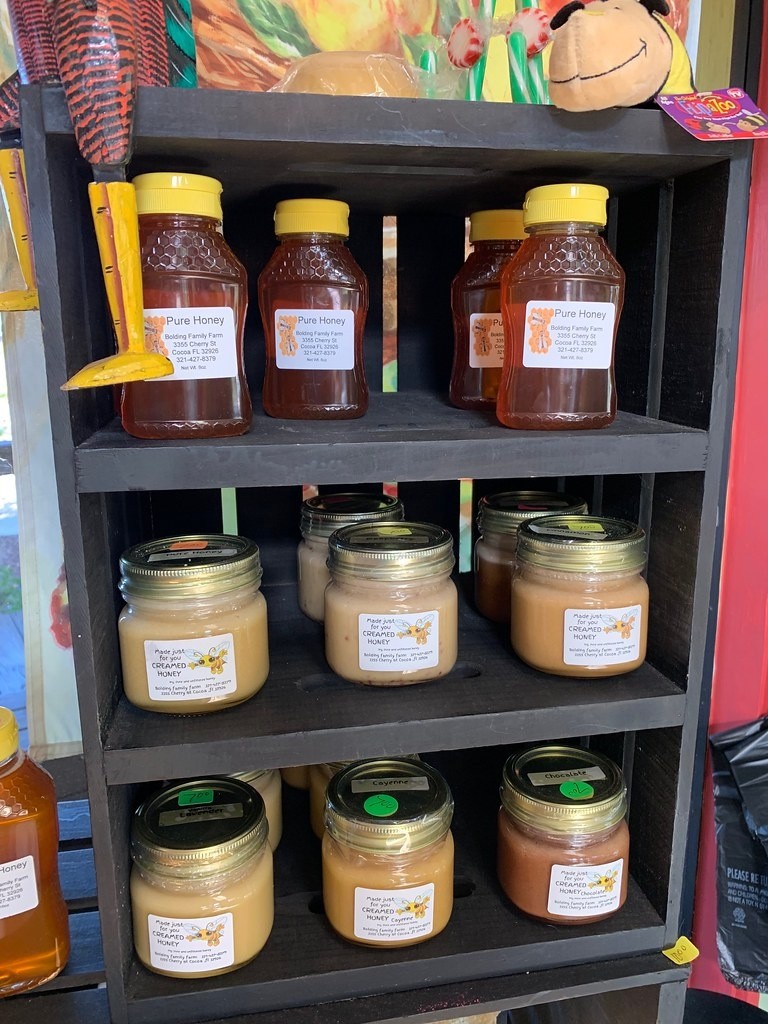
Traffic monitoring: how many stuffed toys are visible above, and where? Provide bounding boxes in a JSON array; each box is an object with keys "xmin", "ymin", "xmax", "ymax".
[{"xmin": 548, "ymin": 0, "xmax": 699, "ymax": 112}]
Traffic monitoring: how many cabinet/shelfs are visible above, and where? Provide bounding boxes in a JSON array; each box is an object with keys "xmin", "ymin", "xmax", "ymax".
[{"xmin": 16, "ymin": 80, "xmax": 755, "ymax": 1023}]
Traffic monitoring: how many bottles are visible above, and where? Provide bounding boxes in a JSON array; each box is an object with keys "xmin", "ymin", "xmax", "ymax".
[
  {"xmin": 494, "ymin": 183, "xmax": 627, "ymax": 430},
  {"xmin": 256, "ymin": 197, "xmax": 371, "ymax": 421},
  {"xmin": 448, "ymin": 209, "xmax": 530, "ymax": 411},
  {"xmin": 0, "ymin": 705, "xmax": 72, "ymax": 1001},
  {"xmin": 119, "ymin": 171, "xmax": 256, "ymax": 442}
]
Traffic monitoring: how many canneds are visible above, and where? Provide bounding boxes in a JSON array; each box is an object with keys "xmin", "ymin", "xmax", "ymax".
[{"xmin": 117, "ymin": 487, "xmax": 649, "ymax": 980}]
